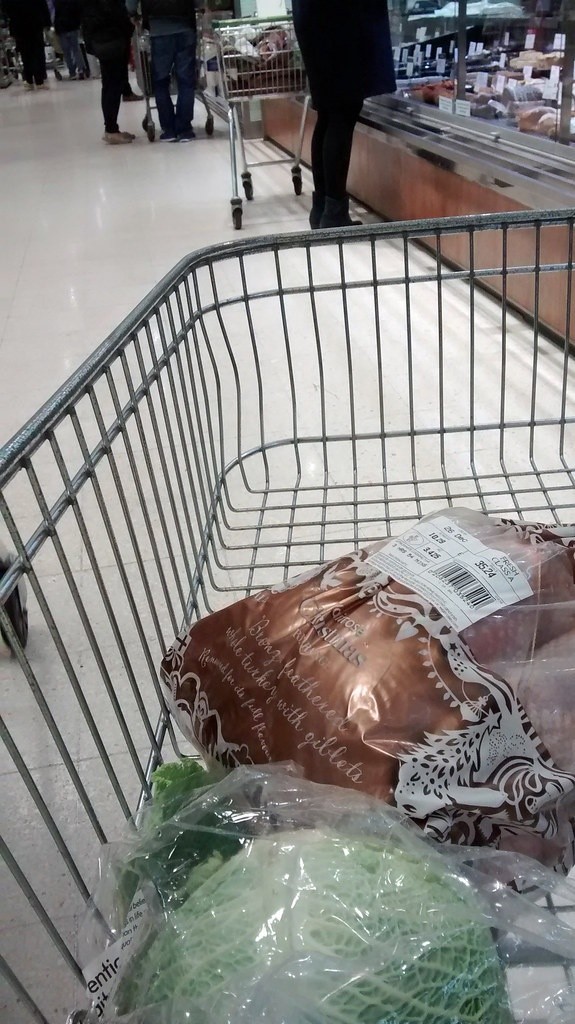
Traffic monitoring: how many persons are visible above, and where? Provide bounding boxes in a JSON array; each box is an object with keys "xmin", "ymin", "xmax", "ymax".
[
  {"xmin": 0, "ymin": 0, "xmax": 203, "ymax": 143},
  {"xmin": 291, "ymin": 0, "xmax": 398, "ymax": 231}
]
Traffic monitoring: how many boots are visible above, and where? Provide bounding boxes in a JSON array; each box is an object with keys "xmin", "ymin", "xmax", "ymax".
[
  {"xmin": 319, "ymin": 194, "xmax": 363, "ymax": 228},
  {"xmin": 308, "ymin": 191, "xmax": 326, "ymax": 230}
]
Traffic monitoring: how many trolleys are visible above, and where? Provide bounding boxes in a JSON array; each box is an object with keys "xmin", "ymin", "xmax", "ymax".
[
  {"xmin": 213, "ymin": 14, "xmax": 314, "ymax": 230},
  {"xmin": 12, "ymin": 32, "xmax": 62, "ymax": 81},
  {"xmin": 130, "ymin": 7, "xmax": 214, "ymax": 142},
  {"xmin": 0, "ymin": 210, "xmax": 575, "ymax": 1024}
]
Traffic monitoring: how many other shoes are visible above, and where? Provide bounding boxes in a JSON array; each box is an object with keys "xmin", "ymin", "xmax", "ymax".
[
  {"xmin": 180, "ymin": 130, "xmax": 196, "ymax": 142},
  {"xmin": 102, "ymin": 131, "xmax": 136, "ymax": 144},
  {"xmin": 68, "ymin": 74, "xmax": 77, "ymax": 81},
  {"xmin": 36, "ymin": 81, "xmax": 49, "ymax": 90},
  {"xmin": 159, "ymin": 130, "xmax": 177, "ymax": 142},
  {"xmin": 78, "ymin": 70, "xmax": 85, "ymax": 80},
  {"xmin": 123, "ymin": 92, "xmax": 144, "ymax": 101},
  {"xmin": 23, "ymin": 80, "xmax": 35, "ymax": 90}
]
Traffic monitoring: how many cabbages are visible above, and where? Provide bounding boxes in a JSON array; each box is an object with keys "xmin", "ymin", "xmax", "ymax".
[{"xmin": 90, "ymin": 756, "xmax": 515, "ymax": 1024}]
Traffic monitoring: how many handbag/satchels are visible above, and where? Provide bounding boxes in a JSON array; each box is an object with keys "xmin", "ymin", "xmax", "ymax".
[
  {"xmin": 157, "ymin": 502, "xmax": 575, "ymax": 942},
  {"xmin": 72, "ymin": 758, "xmax": 575, "ymax": 1024}
]
